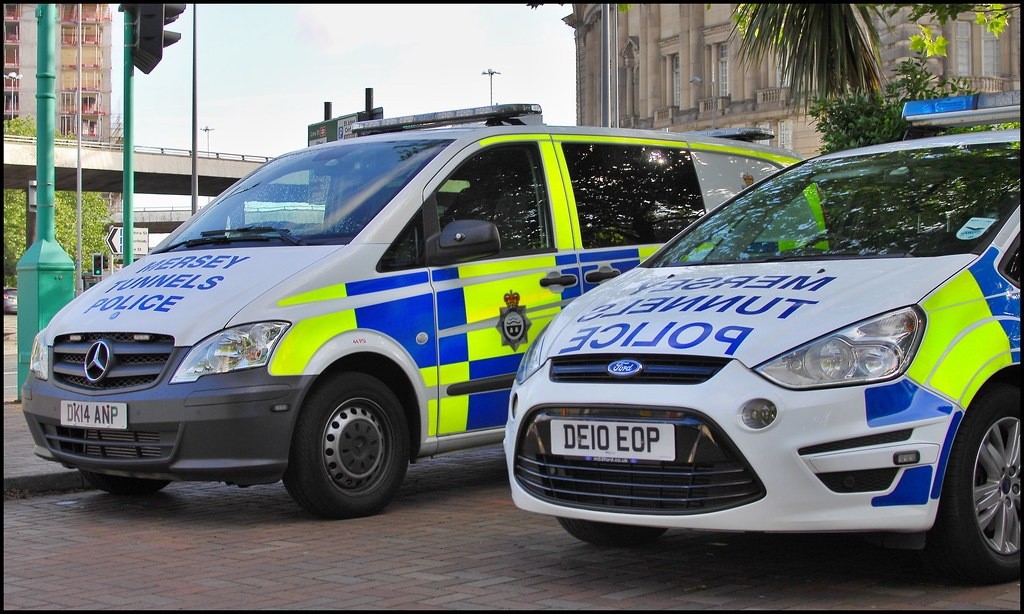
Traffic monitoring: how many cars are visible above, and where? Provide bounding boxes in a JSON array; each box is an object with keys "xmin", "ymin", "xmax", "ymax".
[{"xmin": 6, "ymin": 287, "xmax": 18, "ymax": 314}]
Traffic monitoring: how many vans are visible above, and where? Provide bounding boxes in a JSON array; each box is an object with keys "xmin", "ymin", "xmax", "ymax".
[
  {"xmin": 503, "ymin": 90, "xmax": 1020, "ymax": 584},
  {"xmin": 22, "ymin": 103, "xmax": 830, "ymax": 521}
]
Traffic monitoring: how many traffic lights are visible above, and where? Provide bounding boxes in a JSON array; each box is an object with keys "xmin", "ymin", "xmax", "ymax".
[
  {"xmin": 130, "ymin": 3, "xmax": 188, "ymax": 75},
  {"xmin": 92, "ymin": 254, "xmax": 103, "ymax": 276},
  {"xmin": 103, "ymin": 256, "xmax": 109, "ymax": 270}
]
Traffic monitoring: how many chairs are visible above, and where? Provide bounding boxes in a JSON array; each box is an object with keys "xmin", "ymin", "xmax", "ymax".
[
  {"xmin": 851, "ymin": 167, "xmax": 922, "ymax": 243},
  {"xmin": 335, "ymin": 168, "xmax": 388, "ymax": 231},
  {"xmin": 440, "ymin": 158, "xmax": 531, "ymax": 242}
]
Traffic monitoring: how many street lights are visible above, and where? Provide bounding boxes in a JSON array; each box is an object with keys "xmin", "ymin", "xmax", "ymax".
[
  {"xmin": 199, "ymin": 126, "xmax": 213, "ymax": 155},
  {"xmin": 483, "ymin": 69, "xmax": 501, "ymax": 105},
  {"xmin": 689, "ymin": 78, "xmax": 716, "ymax": 130}
]
